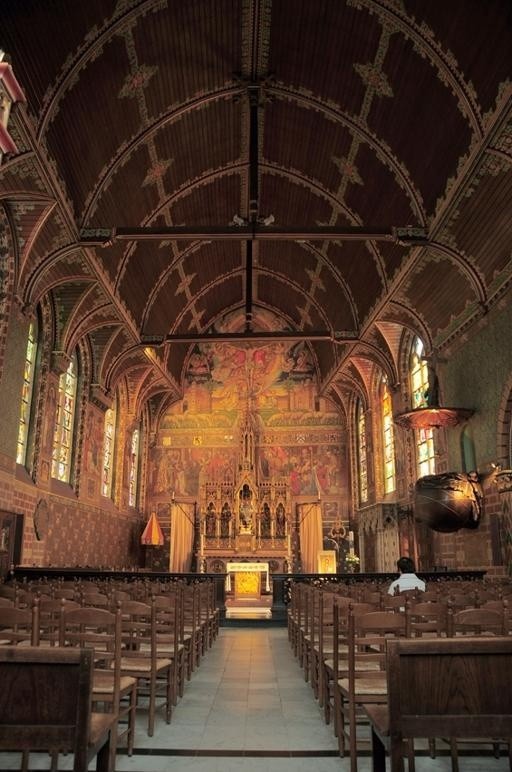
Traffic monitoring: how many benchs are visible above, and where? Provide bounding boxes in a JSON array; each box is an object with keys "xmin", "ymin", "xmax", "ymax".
[
  {"xmin": 1, "ymin": 645, "xmax": 115, "ymax": 770},
  {"xmin": 364, "ymin": 636, "xmax": 512, "ymax": 772}
]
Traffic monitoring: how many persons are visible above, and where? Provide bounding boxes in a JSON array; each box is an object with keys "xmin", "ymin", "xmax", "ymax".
[
  {"xmin": 150, "ymin": 447, "xmax": 341, "ymax": 536},
  {"xmin": 386, "ymin": 555, "xmax": 426, "ymax": 597}
]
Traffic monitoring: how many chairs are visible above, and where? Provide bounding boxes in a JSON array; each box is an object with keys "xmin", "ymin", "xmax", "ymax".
[
  {"xmin": 154, "ymin": 596, "xmax": 191, "ymax": 680},
  {"xmin": 287, "ymin": 579, "xmax": 511, "ymax": 657},
  {"xmin": 304, "ymin": 589, "xmax": 341, "ymax": 680},
  {"xmin": 107, "ymin": 600, "xmax": 172, "ymax": 736},
  {"xmin": 312, "ymin": 591, "xmax": 354, "ymax": 697},
  {"xmin": 168, "ymin": 591, "xmax": 196, "ymax": 672},
  {"xmin": 336, "ymin": 594, "xmax": 412, "ymax": 771},
  {"xmin": 135, "ymin": 596, "xmax": 186, "ymax": 700},
  {"xmin": 3, "ymin": 577, "xmax": 219, "ymax": 666},
  {"xmin": 325, "ymin": 596, "xmax": 386, "ymax": 732},
  {"xmin": 60, "ymin": 606, "xmax": 137, "ymax": 757},
  {"xmin": 317, "ymin": 592, "xmax": 368, "ymax": 725}
]
